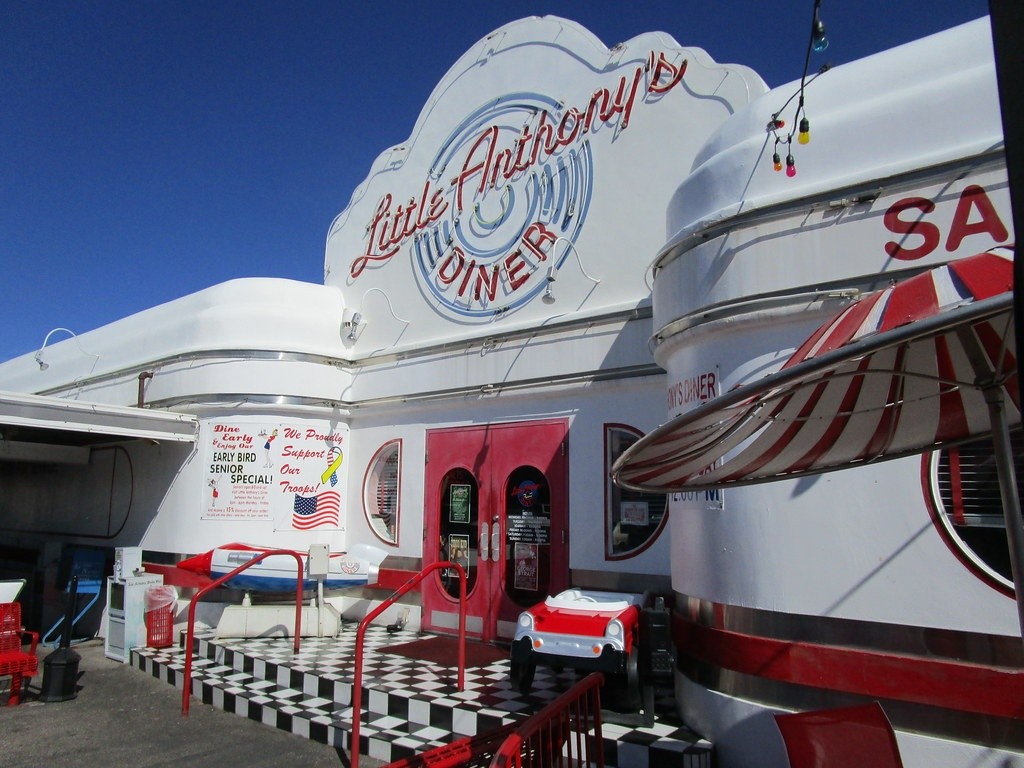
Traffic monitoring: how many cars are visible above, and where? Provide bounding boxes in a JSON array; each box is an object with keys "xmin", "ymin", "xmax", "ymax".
[{"xmin": 507, "ymin": 586, "xmax": 648, "ymax": 697}]
